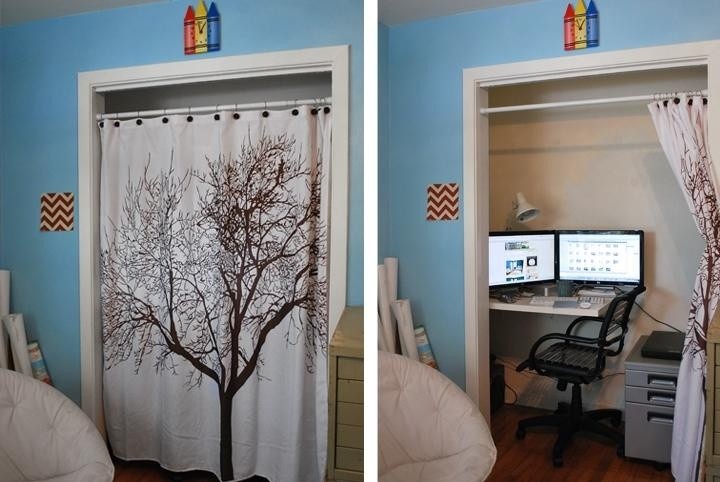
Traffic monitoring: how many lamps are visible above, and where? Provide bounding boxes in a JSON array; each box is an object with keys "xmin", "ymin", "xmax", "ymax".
[{"xmin": 505, "ymin": 191, "xmax": 542, "ymax": 230}]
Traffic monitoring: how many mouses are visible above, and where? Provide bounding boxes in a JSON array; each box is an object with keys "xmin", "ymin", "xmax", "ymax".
[{"xmin": 579, "ymin": 303, "xmax": 591, "ymax": 308}]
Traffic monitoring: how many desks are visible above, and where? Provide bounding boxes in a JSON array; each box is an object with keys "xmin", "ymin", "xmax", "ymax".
[{"xmin": 490, "ymin": 295, "xmax": 614, "ymax": 319}]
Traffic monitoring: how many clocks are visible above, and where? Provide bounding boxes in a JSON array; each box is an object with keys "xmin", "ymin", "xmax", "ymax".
[
  {"xmin": 184, "ymin": 0, "xmax": 220, "ymax": 54},
  {"xmin": 564, "ymin": 0, "xmax": 600, "ymax": 51}
]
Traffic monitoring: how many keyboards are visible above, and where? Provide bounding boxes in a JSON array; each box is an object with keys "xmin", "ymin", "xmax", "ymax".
[{"xmin": 530, "ymin": 296, "xmax": 604, "ymax": 305}]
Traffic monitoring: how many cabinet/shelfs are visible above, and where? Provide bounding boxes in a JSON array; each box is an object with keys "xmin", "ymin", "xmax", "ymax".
[{"xmin": 623, "ymin": 336, "xmax": 681, "ymax": 471}]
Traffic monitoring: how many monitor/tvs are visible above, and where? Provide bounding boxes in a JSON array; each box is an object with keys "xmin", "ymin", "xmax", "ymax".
[
  {"xmin": 556, "ymin": 230, "xmax": 644, "ymax": 296},
  {"xmin": 488, "ymin": 230, "xmax": 555, "ymax": 297}
]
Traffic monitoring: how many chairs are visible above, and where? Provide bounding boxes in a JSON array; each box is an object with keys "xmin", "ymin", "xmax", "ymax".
[
  {"xmin": 378, "ymin": 351, "xmax": 498, "ymax": 481},
  {"xmin": 0, "ymin": 370, "xmax": 117, "ymax": 481},
  {"xmin": 514, "ymin": 286, "xmax": 647, "ymax": 467}
]
